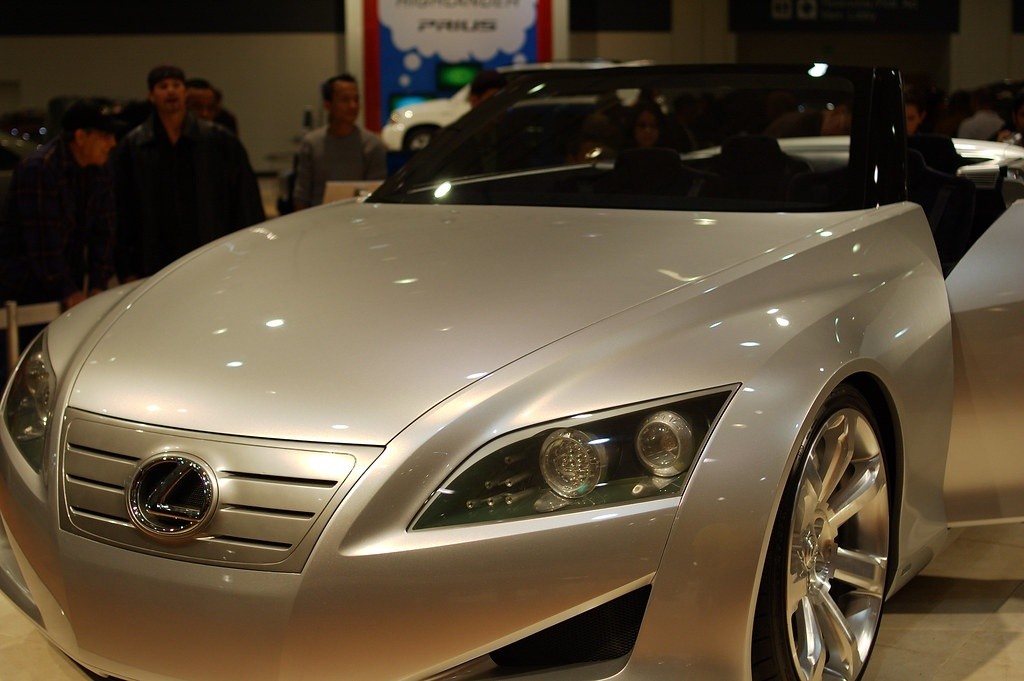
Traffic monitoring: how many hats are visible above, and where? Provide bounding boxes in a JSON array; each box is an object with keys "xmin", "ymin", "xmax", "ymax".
[
  {"xmin": 147, "ymin": 65, "xmax": 185, "ymax": 91},
  {"xmin": 60, "ymin": 97, "xmax": 127, "ymax": 134}
]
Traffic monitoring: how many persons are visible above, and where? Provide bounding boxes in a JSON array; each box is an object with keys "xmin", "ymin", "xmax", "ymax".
[
  {"xmin": 1, "ymin": 65, "xmax": 266, "ymax": 395},
  {"xmin": 950, "ymin": 89, "xmax": 1024, "ymax": 146},
  {"xmin": 294, "ymin": 73, "xmax": 388, "ymax": 210},
  {"xmin": 764, "ymin": 104, "xmax": 851, "ymax": 138},
  {"xmin": 431, "ymin": 70, "xmax": 708, "ymax": 177},
  {"xmin": 904, "ymin": 94, "xmax": 928, "ymax": 134}
]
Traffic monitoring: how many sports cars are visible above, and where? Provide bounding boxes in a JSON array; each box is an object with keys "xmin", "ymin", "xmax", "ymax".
[{"xmin": 0, "ymin": 65, "xmax": 1024, "ymax": 681}]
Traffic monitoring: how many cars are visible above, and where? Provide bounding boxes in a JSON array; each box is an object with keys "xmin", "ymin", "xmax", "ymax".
[{"xmin": 380, "ymin": 68, "xmax": 500, "ymax": 154}]
